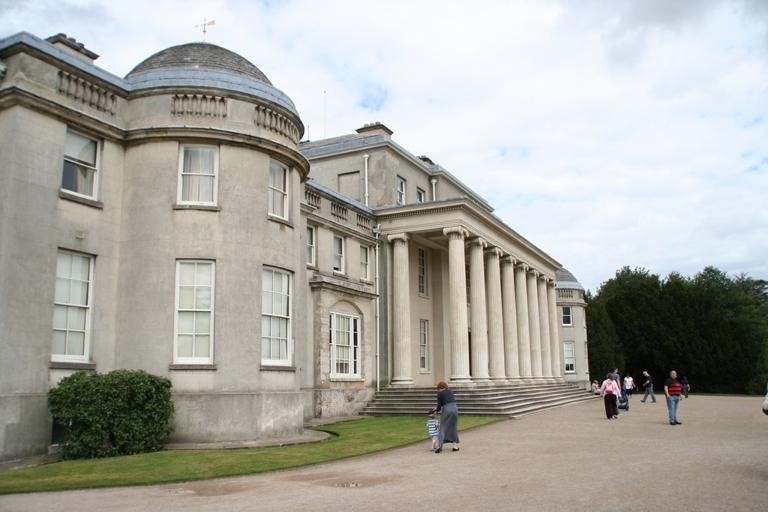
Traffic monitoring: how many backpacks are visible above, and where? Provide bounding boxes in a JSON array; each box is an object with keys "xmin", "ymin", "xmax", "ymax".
[{"xmin": 605, "ymin": 379, "xmax": 615, "ymax": 394}]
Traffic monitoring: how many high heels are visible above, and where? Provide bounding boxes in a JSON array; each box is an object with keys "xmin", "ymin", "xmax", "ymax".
[
  {"xmin": 452, "ymin": 448, "xmax": 459, "ymax": 451},
  {"xmin": 435, "ymin": 448, "xmax": 440, "ymax": 453}
]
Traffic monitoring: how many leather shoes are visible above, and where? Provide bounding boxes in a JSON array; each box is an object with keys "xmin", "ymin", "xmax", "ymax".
[{"xmin": 670, "ymin": 421, "xmax": 681, "ymax": 425}]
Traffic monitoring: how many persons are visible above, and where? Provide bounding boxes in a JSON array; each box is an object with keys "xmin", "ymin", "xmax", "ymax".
[
  {"xmin": 624, "ymin": 372, "xmax": 636, "ymax": 398},
  {"xmin": 640, "ymin": 371, "xmax": 656, "ymax": 403},
  {"xmin": 430, "ymin": 381, "xmax": 460, "ymax": 452},
  {"xmin": 681, "ymin": 375, "xmax": 690, "ymax": 398},
  {"xmin": 609, "ymin": 367, "xmax": 621, "ymax": 389},
  {"xmin": 664, "ymin": 370, "xmax": 682, "ymax": 426},
  {"xmin": 600, "ymin": 373, "xmax": 620, "ymax": 421},
  {"xmin": 425, "ymin": 411, "xmax": 440, "ymax": 451},
  {"xmin": 592, "ymin": 379, "xmax": 600, "ymax": 394}
]
